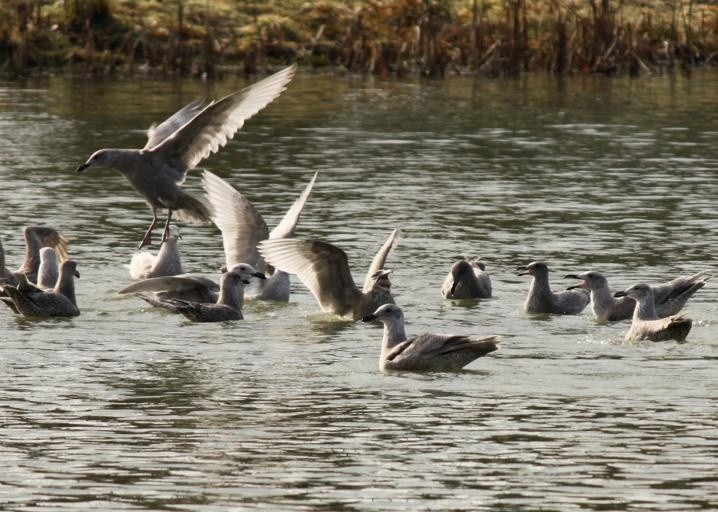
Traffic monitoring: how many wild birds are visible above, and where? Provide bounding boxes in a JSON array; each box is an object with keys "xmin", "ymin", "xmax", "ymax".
[
  {"xmin": 442, "ymin": 261, "xmax": 491, "ymax": 298},
  {"xmin": 3, "ymin": 261, "xmax": 80, "ymax": 318},
  {"xmin": 362, "ymin": 303, "xmax": 500, "ymax": 373},
  {"xmin": 517, "ymin": 262, "xmax": 590, "ymax": 315},
  {"xmin": 118, "ymin": 170, "xmax": 319, "ymax": 322},
  {"xmin": 77, "ymin": 63, "xmax": 298, "ymax": 250},
  {"xmin": 564, "ymin": 272, "xmax": 711, "ymax": 325},
  {"xmin": 0, "ymin": 247, "xmax": 59, "ymax": 314},
  {"xmin": 0, "ymin": 226, "xmax": 70, "ymax": 297},
  {"xmin": 256, "ymin": 228, "xmax": 403, "ymax": 322},
  {"xmin": 613, "ymin": 283, "xmax": 692, "ymax": 342}
]
